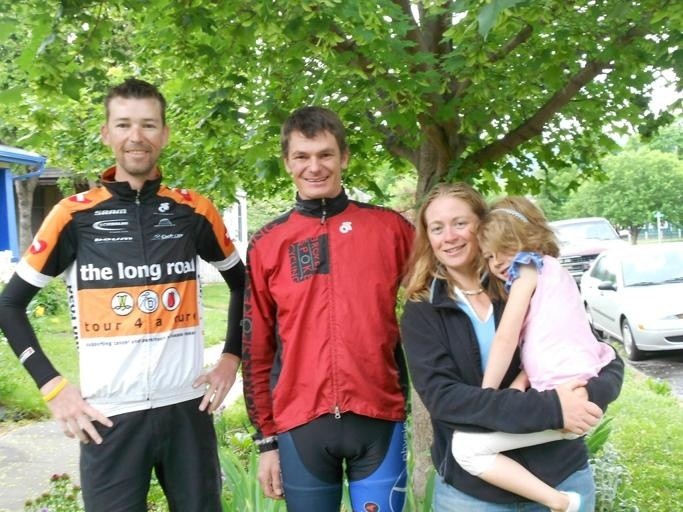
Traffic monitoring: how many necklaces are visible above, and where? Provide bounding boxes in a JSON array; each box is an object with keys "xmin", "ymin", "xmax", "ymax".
[{"xmin": 461, "ymin": 288, "xmax": 487, "ymax": 296}]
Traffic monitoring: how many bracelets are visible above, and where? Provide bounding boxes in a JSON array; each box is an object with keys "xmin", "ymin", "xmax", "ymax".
[{"xmin": 39, "ymin": 378, "xmax": 68, "ymax": 404}]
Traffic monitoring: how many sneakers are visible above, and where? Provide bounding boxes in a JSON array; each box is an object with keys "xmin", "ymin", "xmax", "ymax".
[{"xmin": 551, "ymin": 491, "xmax": 581, "ymax": 511}]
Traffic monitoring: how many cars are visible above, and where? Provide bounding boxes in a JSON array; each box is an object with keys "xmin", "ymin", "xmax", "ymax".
[
  {"xmin": 580, "ymin": 243, "xmax": 683, "ymax": 361},
  {"xmin": 548, "ymin": 217, "xmax": 630, "ymax": 284}
]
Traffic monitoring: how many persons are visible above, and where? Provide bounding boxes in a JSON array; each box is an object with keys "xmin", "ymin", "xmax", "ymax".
[
  {"xmin": 396, "ymin": 180, "xmax": 625, "ymax": 511},
  {"xmin": 1, "ymin": 79, "xmax": 249, "ymax": 512},
  {"xmin": 450, "ymin": 196, "xmax": 618, "ymax": 512},
  {"xmin": 242, "ymin": 105, "xmax": 416, "ymax": 511}
]
